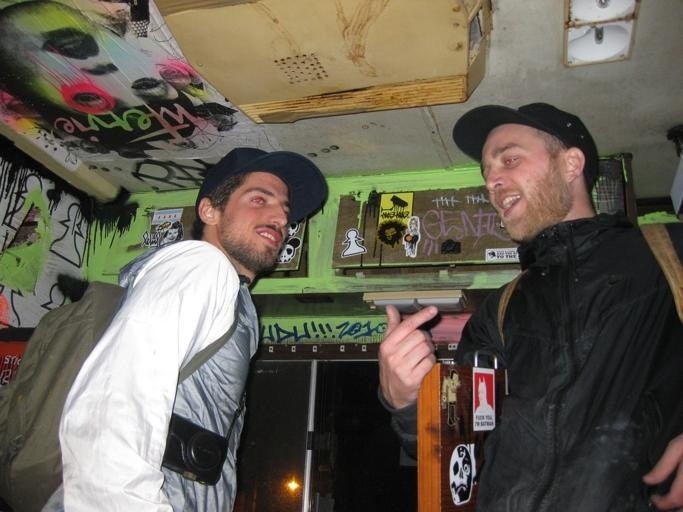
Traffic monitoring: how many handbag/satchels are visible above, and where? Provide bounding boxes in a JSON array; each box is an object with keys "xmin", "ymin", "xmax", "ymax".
[{"xmin": 0, "ymin": 280, "xmax": 127, "ymax": 511}]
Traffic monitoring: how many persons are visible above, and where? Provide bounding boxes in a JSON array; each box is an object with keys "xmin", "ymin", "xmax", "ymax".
[
  {"xmin": 640, "ymin": 432, "xmax": 683, "ymax": 512},
  {"xmin": 39, "ymin": 148, "xmax": 329, "ymax": 512},
  {"xmin": 375, "ymin": 103, "xmax": 683, "ymax": 512}
]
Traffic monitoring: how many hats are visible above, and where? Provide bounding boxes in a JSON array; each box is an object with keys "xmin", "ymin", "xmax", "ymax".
[
  {"xmin": 195, "ymin": 148, "xmax": 328, "ymax": 224},
  {"xmin": 453, "ymin": 103, "xmax": 599, "ymax": 188}
]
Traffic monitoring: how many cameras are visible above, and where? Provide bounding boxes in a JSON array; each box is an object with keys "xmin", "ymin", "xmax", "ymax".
[{"xmin": 162, "ymin": 413, "xmax": 228, "ymax": 485}]
